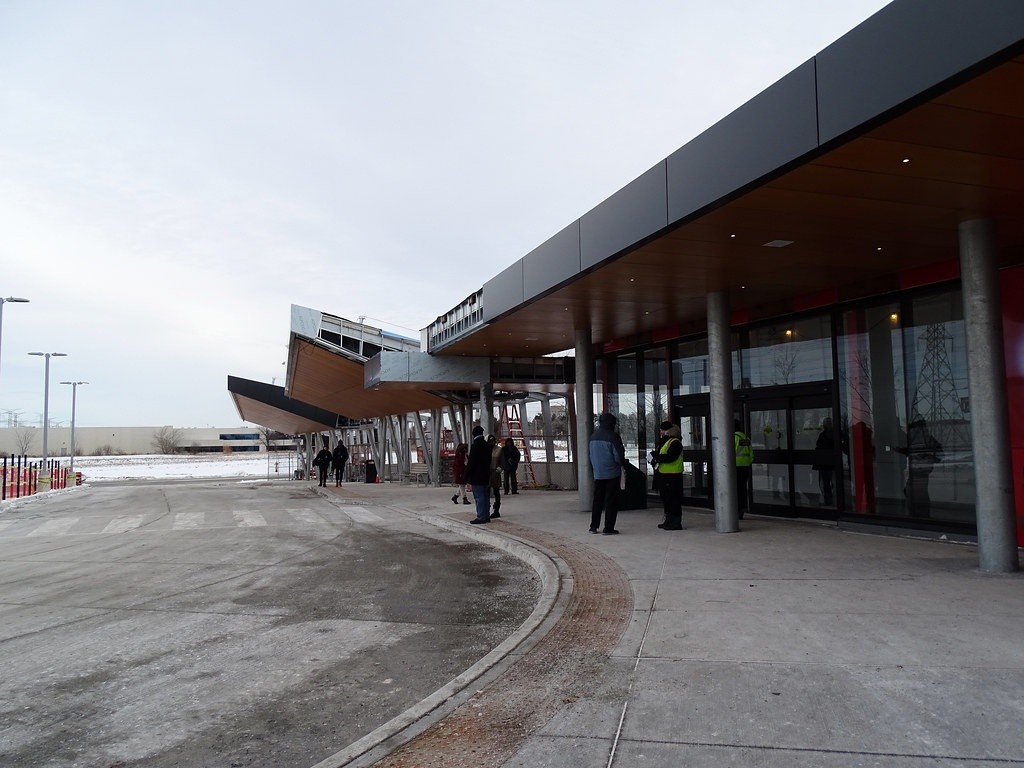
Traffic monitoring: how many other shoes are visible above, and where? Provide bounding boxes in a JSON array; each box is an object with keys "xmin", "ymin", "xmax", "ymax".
[
  {"xmin": 589, "ymin": 525, "xmax": 597, "ymax": 534},
  {"xmin": 739, "ymin": 511, "xmax": 743, "ymax": 520},
  {"xmin": 504, "ymin": 491, "xmax": 508, "ymax": 495},
  {"xmin": 318, "ymin": 484, "xmax": 322, "ymax": 486},
  {"xmin": 664, "ymin": 522, "xmax": 682, "ymax": 530},
  {"xmin": 819, "ymin": 502, "xmax": 834, "ymax": 506},
  {"xmin": 603, "ymin": 528, "xmax": 619, "ymax": 535},
  {"xmin": 658, "ymin": 520, "xmax": 671, "ymax": 528},
  {"xmin": 512, "ymin": 492, "xmax": 519, "ymax": 494},
  {"xmin": 470, "ymin": 519, "xmax": 487, "ymax": 524},
  {"xmin": 490, "ymin": 512, "xmax": 500, "ymax": 518},
  {"xmin": 324, "ymin": 484, "xmax": 326, "ymax": 487},
  {"xmin": 487, "ymin": 517, "xmax": 490, "ymax": 522}
]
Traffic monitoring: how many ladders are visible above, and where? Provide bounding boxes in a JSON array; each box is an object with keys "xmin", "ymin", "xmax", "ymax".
[{"xmin": 496, "ymin": 404, "xmax": 537, "ymax": 488}]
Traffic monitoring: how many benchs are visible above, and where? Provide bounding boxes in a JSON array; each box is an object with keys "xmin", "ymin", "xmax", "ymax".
[{"xmin": 385, "ymin": 464, "xmax": 429, "ymax": 488}]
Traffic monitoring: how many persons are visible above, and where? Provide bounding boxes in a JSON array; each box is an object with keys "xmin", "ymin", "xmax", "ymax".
[
  {"xmin": 893, "ymin": 419, "xmax": 945, "ymax": 518},
  {"xmin": 647, "ymin": 421, "xmax": 684, "ymax": 531},
  {"xmin": 811, "ymin": 414, "xmax": 846, "ymax": 506},
  {"xmin": 732, "ymin": 419, "xmax": 754, "ymax": 520},
  {"xmin": 312, "ymin": 444, "xmax": 332, "ymax": 487},
  {"xmin": 502, "ymin": 438, "xmax": 520, "ymax": 495},
  {"xmin": 588, "ymin": 412, "xmax": 629, "ymax": 535},
  {"xmin": 451, "ymin": 442, "xmax": 473, "ymax": 505},
  {"xmin": 485, "ymin": 434, "xmax": 506, "ymax": 519},
  {"xmin": 466, "ymin": 426, "xmax": 493, "ymax": 524},
  {"xmin": 842, "ymin": 421, "xmax": 877, "ymax": 514},
  {"xmin": 331, "ymin": 440, "xmax": 349, "ymax": 487}
]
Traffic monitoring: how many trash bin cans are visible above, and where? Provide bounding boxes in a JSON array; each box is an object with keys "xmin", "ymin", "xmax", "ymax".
[
  {"xmin": 294, "ymin": 470, "xmax": 303, "ymax": 480},
  {"xmin": 364, "ymin": 460, "xmax": 377, "ymax": 484}
]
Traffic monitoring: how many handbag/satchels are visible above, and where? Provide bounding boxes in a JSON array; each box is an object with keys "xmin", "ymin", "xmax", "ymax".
[
  {"xmin": 620, "ymin": 461, "xmax": 647, "ymax": 492},
  {"xmin": 652, "ymin": 463, "xmax": 660, "ymax": 493}
]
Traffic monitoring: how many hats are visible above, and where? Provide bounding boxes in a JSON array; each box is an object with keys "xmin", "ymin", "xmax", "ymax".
[
  {"xmin": 660, "ymin": 421, "xmax": 673, "ymax": 430},
  {"xmin": 472, "ymin": 426, "xmax": 484, "ymax": 436}
]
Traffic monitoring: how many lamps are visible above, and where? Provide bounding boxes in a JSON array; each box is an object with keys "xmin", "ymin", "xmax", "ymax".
[
  {"xmin": 769, "ymin": 327, "xmax": 795, "ymax": 338},
  {"xmin": 869, "ymin": 313, "xmax": 898, "ymax": 331}
]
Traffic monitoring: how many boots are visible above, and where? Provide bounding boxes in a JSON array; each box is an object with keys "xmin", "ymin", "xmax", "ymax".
[
  {"xmin": 452, "ymin": 494, "xmax": 459, "ymax": 504},
  {"xmin": 339, "ymin": 481, "xmax": 342, "ymax": 487},
  {"xmin": 463, "ymin": 497, "xmax": 471, "ymax": 504},
  {"xmin": 336, "ymin": 481, "xmax": 338, "ymax": 487}
]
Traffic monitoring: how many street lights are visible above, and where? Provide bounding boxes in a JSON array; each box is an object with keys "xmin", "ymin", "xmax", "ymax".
[
  {"xmin": 0, "ymin": 296, "xmax": 30, "ymax": 370},
  {"xmin": 27, "ymin": 351, "xmax": 68, "ymax": 493},
  {"xmin": 59, "ymin": 381, "xmax": 89, "ymax": 486}
]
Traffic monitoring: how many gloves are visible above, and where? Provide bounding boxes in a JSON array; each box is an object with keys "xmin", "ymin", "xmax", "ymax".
[{"xmin": 650, "ymin": 450, "xmax": 661, "ymax": 467}]
